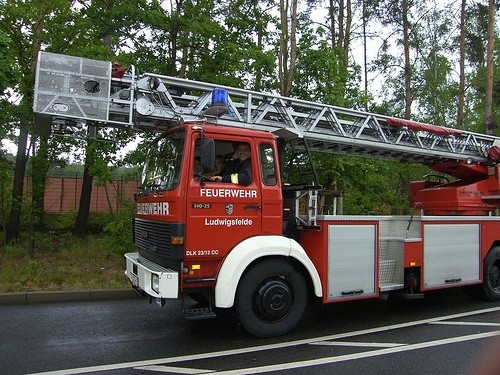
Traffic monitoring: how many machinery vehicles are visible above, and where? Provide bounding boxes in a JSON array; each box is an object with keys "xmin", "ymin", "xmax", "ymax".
[{"xmin": 32, "ymin": 47, "xmax": 499, "ymax": 340}]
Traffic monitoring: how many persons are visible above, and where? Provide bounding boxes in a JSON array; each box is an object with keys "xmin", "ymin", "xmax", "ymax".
[
  {"xmin": 211, "ymin": 143, "xmax": 252, "ymax": 186},
  {"xmin": 193, "ymin": 155, "xmax": 226, "ymax": 182}
]
